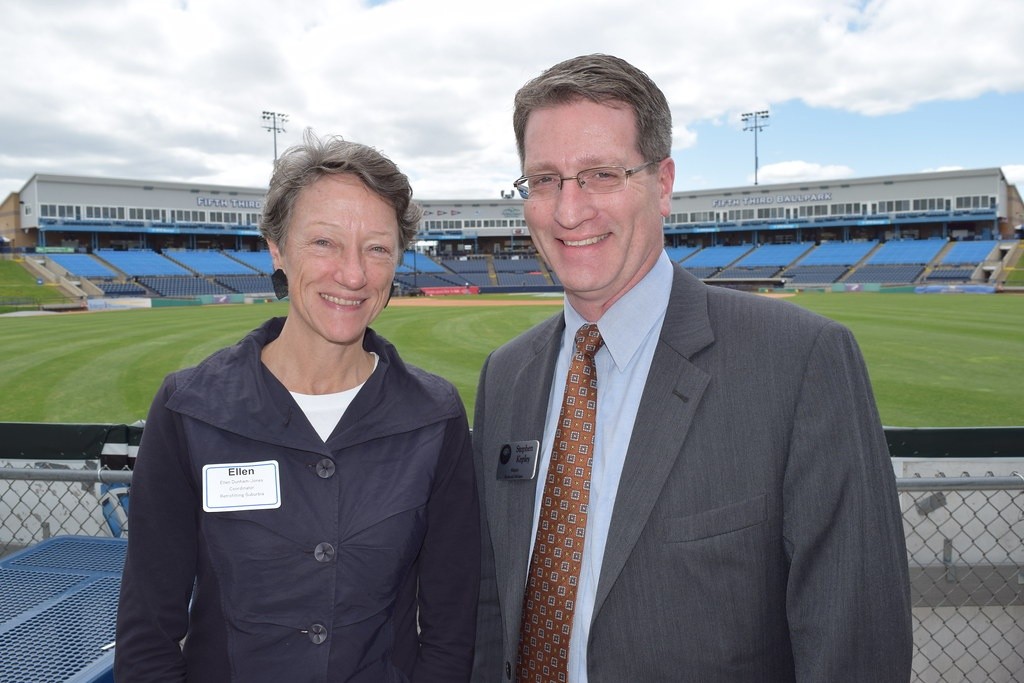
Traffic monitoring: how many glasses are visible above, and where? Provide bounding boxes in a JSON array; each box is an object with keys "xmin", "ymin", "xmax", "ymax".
[{"xmin": 515, "ymin": 161, "xmax": 664, "ymax": 202}]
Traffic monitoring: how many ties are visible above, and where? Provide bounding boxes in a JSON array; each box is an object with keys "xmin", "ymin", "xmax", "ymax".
[{"xmin": 516, "ymin": 324, "xmax": 605, "ymax": 683}]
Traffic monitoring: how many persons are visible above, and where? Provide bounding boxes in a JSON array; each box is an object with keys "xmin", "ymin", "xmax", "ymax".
[
  {"xmin": 472, "ymin": 54, "xmax": 915, "ymax": 682},
  {"xmin": 112, "ymin": 129, "xmax": 483, "ymax": 682}
]
